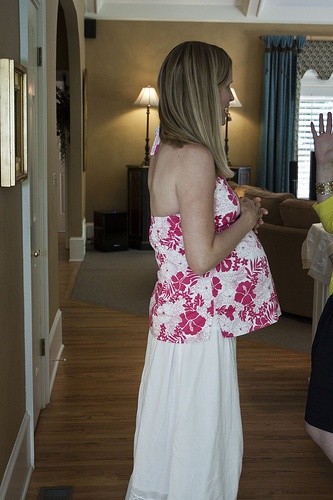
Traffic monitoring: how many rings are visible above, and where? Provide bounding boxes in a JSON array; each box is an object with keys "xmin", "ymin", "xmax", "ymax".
[{"xmin": 319, "ymin": 131, "xmax": 324, "ymax": 134}]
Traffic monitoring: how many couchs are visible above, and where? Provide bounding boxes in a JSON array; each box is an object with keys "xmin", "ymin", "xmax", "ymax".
[{"xmin": 229, "ymin": 182, "xmax": 321, "ymax": 318}]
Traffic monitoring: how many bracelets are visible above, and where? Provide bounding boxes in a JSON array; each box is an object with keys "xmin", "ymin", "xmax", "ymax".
[{"xmin": 315, "ymin": 181, "xmax": 333, "ymax": 195}]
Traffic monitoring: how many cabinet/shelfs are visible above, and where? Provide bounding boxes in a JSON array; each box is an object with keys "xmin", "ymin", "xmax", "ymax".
[{"xmin": 126, "ymin": 165, "xmax": 252, "ymax": 249}]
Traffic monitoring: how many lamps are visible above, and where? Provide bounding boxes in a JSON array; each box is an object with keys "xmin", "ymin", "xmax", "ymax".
[
  {"xmin": 132, "ymin": 87, "xmax": 159, "ymax": 166},
  {"xmin": 224, "ymin": 88, "xmax": 243, "ymax": 166}
]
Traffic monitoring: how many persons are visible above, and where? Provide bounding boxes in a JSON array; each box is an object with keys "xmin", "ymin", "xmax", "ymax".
[
  {"xmin": 304, "ymin": 112, "xmax": 333, "ymax": 434},
  {"xmin": 124, "ymin": 41, "xmax": 282, "ymax": 500}
]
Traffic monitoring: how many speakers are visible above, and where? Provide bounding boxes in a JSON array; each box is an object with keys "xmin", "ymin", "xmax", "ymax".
[
  {"xmin": 84, "ymin": 18, "xmax": 96, "ymax": 38},
  {"xmin": 93, "ymin": 211, "xmax": 128, "ymax": 252}
]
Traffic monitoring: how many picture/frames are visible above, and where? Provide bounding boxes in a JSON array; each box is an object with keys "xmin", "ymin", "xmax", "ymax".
[{"xmin": 0, "ymin": 59, "xmax": 29, "ymax": 187}]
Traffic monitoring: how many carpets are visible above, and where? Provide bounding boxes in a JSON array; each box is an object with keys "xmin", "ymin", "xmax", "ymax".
[{"xmin": 71, "ymin": 239, "xmax": 313, "ymax": 355}]
